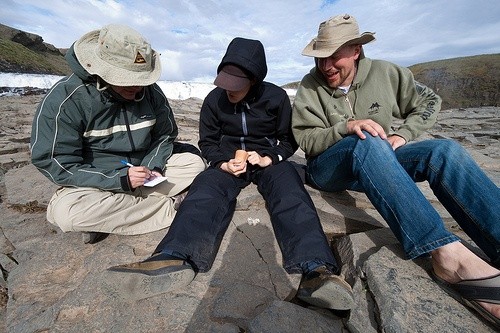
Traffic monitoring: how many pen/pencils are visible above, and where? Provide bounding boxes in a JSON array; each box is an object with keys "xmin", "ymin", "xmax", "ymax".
[{"xmin": 120, "ymin": 159, "xmax": 153, "ymax": 176}]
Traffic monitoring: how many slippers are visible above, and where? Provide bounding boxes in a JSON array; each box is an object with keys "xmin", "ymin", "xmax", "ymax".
[{"xmin": 430, "ymin": 265, "xmax": 500, "ymax": 330}]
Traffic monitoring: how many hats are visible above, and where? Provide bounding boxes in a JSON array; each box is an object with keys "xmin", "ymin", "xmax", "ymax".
[
  {"xmin": 301, "ymin": 15, "xmax": 375, "ymax": 58},
  {"xmin": 214, "ymin": 64, "xmax": 250, "ymax": 91},
  {"xmin": 73, "ymin": 25, "xmax": 161, "ymax": 86}
]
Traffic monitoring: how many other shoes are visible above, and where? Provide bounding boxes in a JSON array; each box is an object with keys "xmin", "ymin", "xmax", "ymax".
[
  {"xmin": 106, "ymin": 252, "xmax": 196, "ymax": 300},
  {"xmin": 82, "ymin": 232, "xmax": 108, "ymax": 244},
  {"xmin": 172, "ymin": 191, "xmax": 189, "ymax": 210},
  {"xmin": 296, "ymin": 265, "xmax": 354, "ymax": 309}
]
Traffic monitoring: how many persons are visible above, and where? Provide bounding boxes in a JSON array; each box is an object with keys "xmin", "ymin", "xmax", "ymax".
[
  {"xmin": 30, "ymin": 23, "xmax": 206, "ymax": 244},
  {"xmin": 291, "ymin": 13, "xmax": 500, "ymax": 327},
  {"xmin": 104, "ymin": 37, "xmax": 357, "ymax": 309}
]
